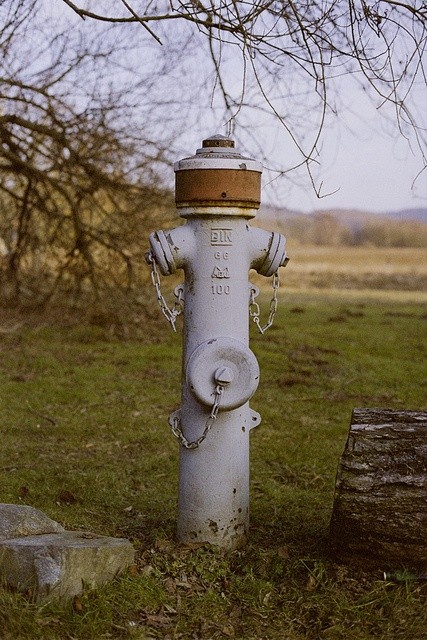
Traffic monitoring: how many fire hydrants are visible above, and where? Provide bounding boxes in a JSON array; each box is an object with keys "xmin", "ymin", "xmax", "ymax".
[{"xmin": 144, "ymin": 134, "xmax": 290, "ymax": 553}]
